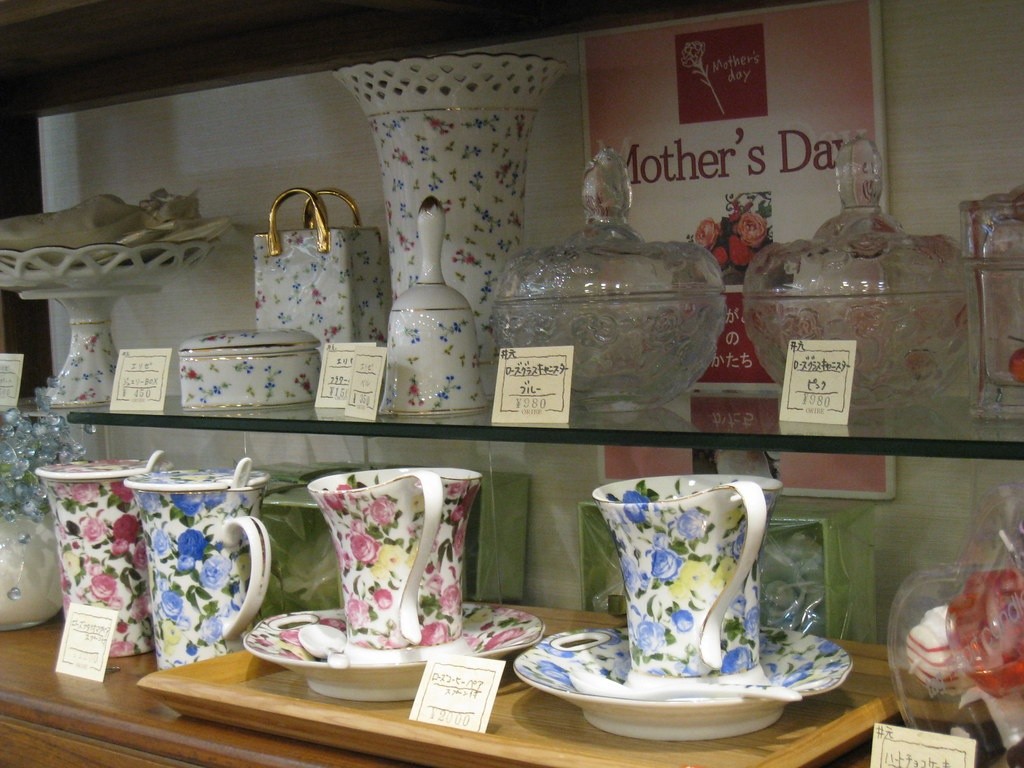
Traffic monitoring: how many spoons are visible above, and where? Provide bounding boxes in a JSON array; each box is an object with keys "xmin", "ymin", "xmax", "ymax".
[
  {"xmin": 298, "ymin": 624, "xmax": 350, "ymax": 669},
  {"xmin": 567, "ymin": 668, "xmax": 803, "ymax": 702}
]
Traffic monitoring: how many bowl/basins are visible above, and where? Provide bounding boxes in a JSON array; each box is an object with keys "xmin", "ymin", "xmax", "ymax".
[
  {"xmin": 487, "ymin": 141, "xmax": 725, "ymax": 414},
  {"xmin": 742, "ymin": 134, "xmax": 964, "ymax": 407}
]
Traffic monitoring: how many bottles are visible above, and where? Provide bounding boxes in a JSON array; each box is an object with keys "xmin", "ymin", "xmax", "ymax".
[{"xmin": 378, "ymin": 195, "xmax": 488, "ymax": 417}]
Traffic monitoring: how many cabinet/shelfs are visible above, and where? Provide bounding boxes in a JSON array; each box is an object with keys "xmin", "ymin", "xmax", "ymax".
[{"xmin": 0, "ymin": 393, "xmax": 1024, "ymax": 768}]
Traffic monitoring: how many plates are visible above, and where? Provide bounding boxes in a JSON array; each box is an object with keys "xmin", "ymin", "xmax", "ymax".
[
  {"xmin": 513, "ymin": 624, "xmax": 853, "ymax": 741},
  {"xmin": 243, "ymin": 604, "xmax": 546, "ymax": 702}
]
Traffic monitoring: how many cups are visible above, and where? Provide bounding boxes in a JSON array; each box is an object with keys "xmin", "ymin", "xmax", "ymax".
[
  {"xmin": 591, "ymin": 474, "xmax": 785, "ymax": 685},
  {"xmin": 123, "ymin": 469, "xmax": 272, "ymax": 672},
  {"xmin": 35, "ymin": 457, "xmax": 177, "ymax": 656},
  {"xmin": 306, "ymin": 468, "xmax": 483, "ymax": 664},
  {"xmin": 958, "ymin": 190, "xmax": 1024, "ymax": 421}
]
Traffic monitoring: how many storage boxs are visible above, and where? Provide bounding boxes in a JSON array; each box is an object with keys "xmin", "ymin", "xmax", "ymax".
[
  {"xmin": 233, "ymin": 465, "xmax": 531, "ymax": 621},
  {"xmin": 578, "ymin": 497, "xmax": 879, "ymax": 642}
]
